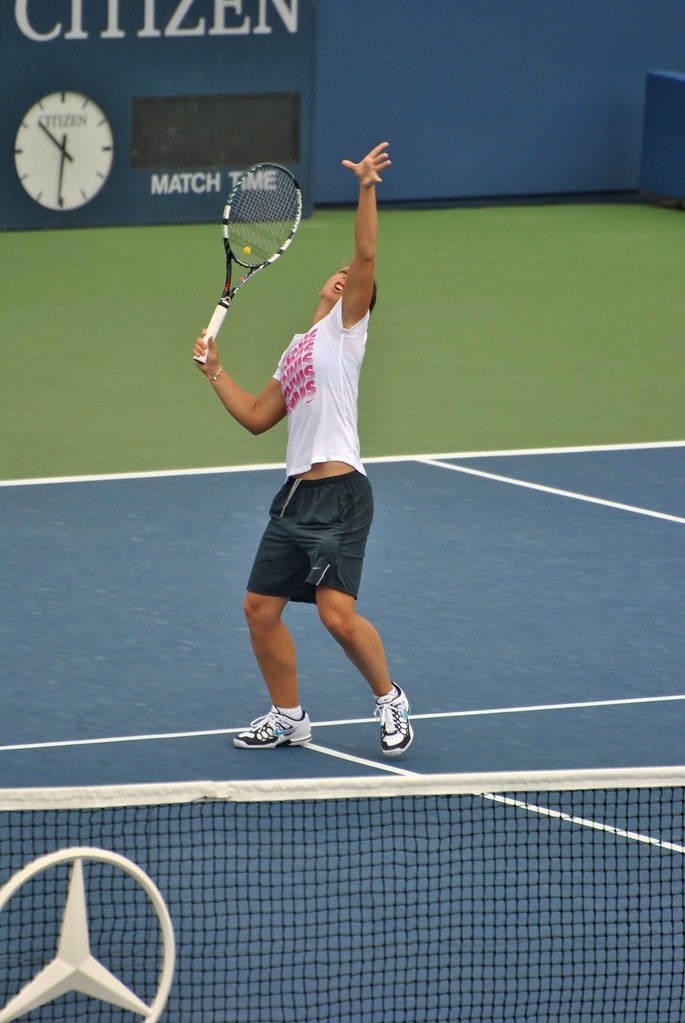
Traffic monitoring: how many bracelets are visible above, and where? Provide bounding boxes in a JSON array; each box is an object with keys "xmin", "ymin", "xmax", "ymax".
[{"xmin": 201, "ymin": 364, "xmax": 222, "ymax": 381}]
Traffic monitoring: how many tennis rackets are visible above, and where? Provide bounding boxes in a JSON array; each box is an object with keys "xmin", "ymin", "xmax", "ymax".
[{"xmin": 193, "ymin": 162, "xmax": 303, "ymax": 366}]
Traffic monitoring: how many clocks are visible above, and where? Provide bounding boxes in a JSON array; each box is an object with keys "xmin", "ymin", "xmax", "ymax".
[{"xmin": 12, "ymin": 89, "xmax": 117, "ymax": 213}]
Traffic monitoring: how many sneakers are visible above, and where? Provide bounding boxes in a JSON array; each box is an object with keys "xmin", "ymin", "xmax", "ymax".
[
  {"xmin": 232, "ymin": 705, "xmax": 313, "ymax": 748},
  {"xmin": 374, "ymin": 681, "xmax": 414, "ymax": 757}
]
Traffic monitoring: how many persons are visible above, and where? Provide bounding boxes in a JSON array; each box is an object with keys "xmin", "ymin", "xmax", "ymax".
[{"xmin": 191, "ymin": 142, "xmax": 414, "ymax": 756}]
